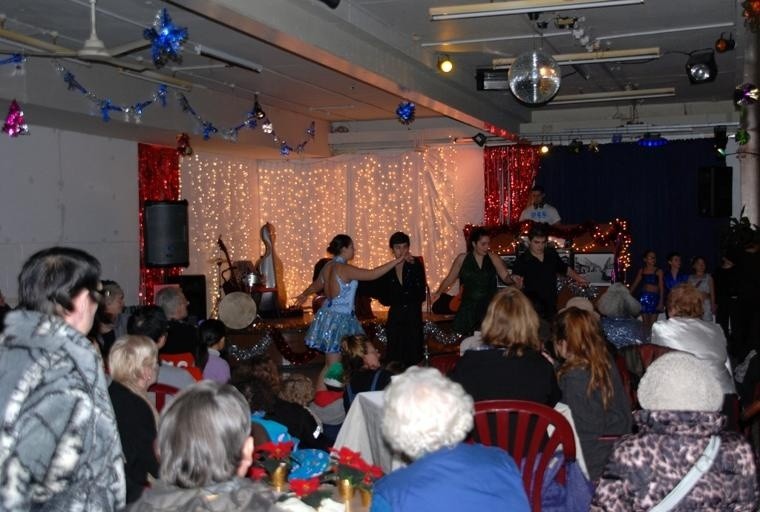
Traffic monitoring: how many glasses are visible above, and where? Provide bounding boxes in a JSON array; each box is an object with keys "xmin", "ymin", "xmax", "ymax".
[{"xmin": 85, "ymin": 284, "xmax": 111, "ymax": 303}]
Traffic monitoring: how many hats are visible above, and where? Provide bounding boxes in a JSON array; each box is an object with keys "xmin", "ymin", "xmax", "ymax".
[
  {"xmin": 598, "ymin": 282, "xmax": 641, "ymax": 318},
  {"xmin": 558, "ymin": 296, "xmax": 600, "ymax": 321}
]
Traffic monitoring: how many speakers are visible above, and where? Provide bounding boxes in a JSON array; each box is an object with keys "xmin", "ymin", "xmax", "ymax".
[
  {"xmin": 168, "ymin": 275, "xmax": 206, "ymax": 323},
  {"xmin": 477, "ymin": 69, "xmax": 510, "ymax": 91},
  {"xmin": 144, "ymin": 200, "xmax": 189, "ymax": 267},
  {"xmin": 698, "ymin": 166, "xmax": 732, "ymax": 218}
]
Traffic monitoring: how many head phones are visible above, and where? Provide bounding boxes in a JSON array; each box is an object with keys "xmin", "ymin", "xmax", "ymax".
[{"xmin": 533, "ymin": 200, "xmax": 546, "ymax": 209}]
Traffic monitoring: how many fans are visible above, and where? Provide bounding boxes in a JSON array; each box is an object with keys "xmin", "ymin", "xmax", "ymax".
[{"xmin": 0, "ymin": 0, "xmax": 158, "ymax": 75}]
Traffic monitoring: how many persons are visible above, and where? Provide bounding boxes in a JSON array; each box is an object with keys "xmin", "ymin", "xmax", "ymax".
[
  {"xmin": 130, "ymin": 379, "xmax": 280, "ymax": 512},
  {"xmin": 236, "ymin": 335, "xmax": 398, "ymax": 448},
  {"xmin": 376, "ymin": 232, "xmax": 427, "ymax": 367},
  {"xmin": 126, "ymin": 305, "xmax": 195, "ymax": 411},
  {"xmin": 157, "ymin": 286, "xmax": 195, "ymax": 354},
  {"xmin": 431, "ymin": 228, "xmax": 520, "ymax": 336},
  {"xmin": 450, "ymin": 290, "xmax": 563, "ymax": 456},
  {"xmin": 0, "ymin": 246, "xmax": 126, "ymax": 512},
  {"xmin": 369, "ymin": 368, "xmax": 532, "ymax": 512},
  {"xmin": 291, "ymin": 234, "xmax": 414, "ymax": 394},
  {"xmin": 514, "ymin": 230, "xmax": 588, "ymax": 347},
  {"xmin": 630, "ymin": 228, "xmax": 760, "ymax": 359},
  {"xmin": 558, "ymin": 283, "xmax": 760, "ymax": 459},
  {"xmin": 553, "ymin": 308, "xmax": 631, "ymax": 478},
  {"xmin": 591, "ymin": 351, "xmax": 760, "ymax": 512},
  {"xmin": 107, "ymin": 335, "xmax": 162, "ymax": 504},
  {"xmin": 518, "ymin": 185, "xmax": 562, "ymax": 226},
  {"xmin": 93, "ymin": 280, "xmax": 124, "ymax": 355},
  {"xmin": 193, "ymin": 319, "xmax": 230, "ymax": 386}
]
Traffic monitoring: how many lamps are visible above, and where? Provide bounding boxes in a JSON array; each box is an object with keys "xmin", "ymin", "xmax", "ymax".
[
  {"xmin": 192, "ymin": 41, "xmax": 265, "ymax": 77},
  {"xmin": 424, "ymin": 0, "xmax": 734, "ymax": 110},
  {"xmin": 0, "ymin": 28, "xmax": 205, "ymax": 98},
  {"xmin": 538, "ymin": 128, "xmax": 675, "ymax": 155}
]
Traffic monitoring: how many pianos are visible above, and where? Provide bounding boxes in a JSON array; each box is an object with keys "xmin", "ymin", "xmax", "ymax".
[{"xmin": 471, "ymin": 224, "xmax": 559, "ymax": 240}]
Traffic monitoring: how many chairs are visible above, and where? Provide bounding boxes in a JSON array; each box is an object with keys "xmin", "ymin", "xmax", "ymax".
[
  {"xmin": 609, "ymin": 344, "xmax": 676, "ymax": 415},
  {"xmin": 146, "ymin": 384, "xmax": 180, "ymax": 412},
  {"xmin": 419, "ymin": 354, "xmax": 459, "ymax": 379},
  {"xmin": 462, "ymin": 399, "xmax": 577, "ymax": 511}
]
